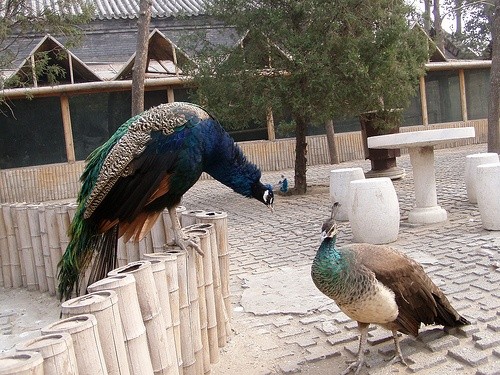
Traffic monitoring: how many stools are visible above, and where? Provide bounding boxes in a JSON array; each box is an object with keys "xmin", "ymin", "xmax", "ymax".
[
  {"xmin": 474, "ymin": 162, "xmax": 500, "ymax": 231},
  {"xmin": 330, "ymin": 167, "xmax": 366, "ymax": 223},
  {"xmin": 463, "ymin": 153, "xmax": 500, "ymax": 203},
  {"xmin": 348, "ymin": 177, "xmax": 400, "ymax": 245}
]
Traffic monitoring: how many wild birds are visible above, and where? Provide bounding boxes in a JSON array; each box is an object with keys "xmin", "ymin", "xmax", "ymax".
[
  {"xmin": 55, "ymin": 102, "xmax": 289, "ymax": 319},
  {"xmin": 310, "ymin": 202, "xmax": 472, "ymax": 375}
]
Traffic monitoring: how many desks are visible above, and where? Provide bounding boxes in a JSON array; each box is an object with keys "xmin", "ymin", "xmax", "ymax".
[{"xmin": 367, "ymin": 126, "xmax": 476, "ymax": 222}]
